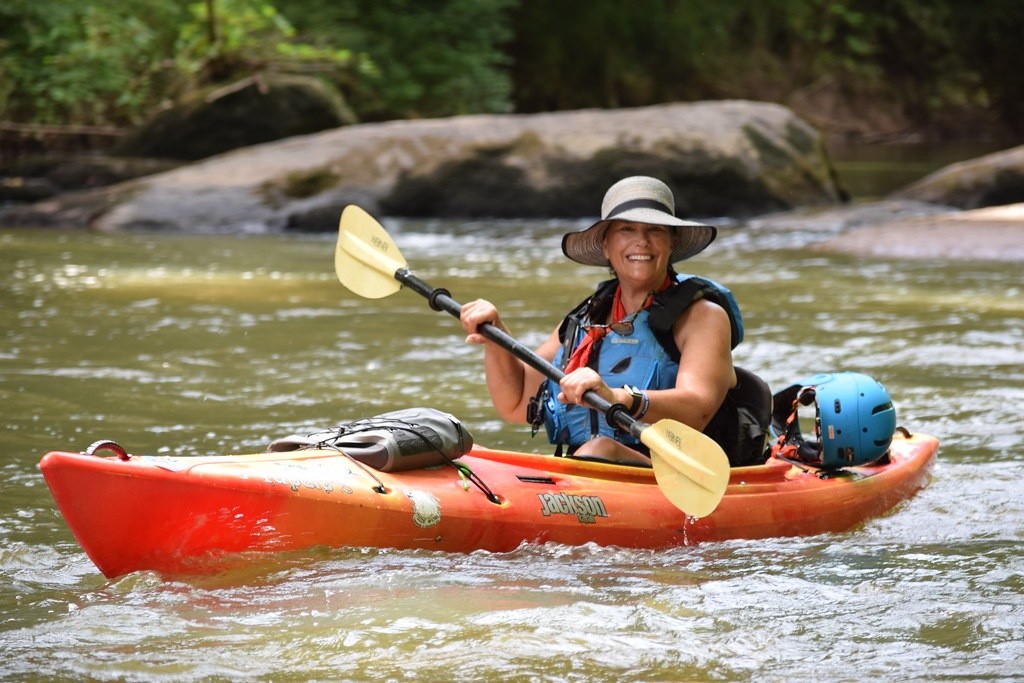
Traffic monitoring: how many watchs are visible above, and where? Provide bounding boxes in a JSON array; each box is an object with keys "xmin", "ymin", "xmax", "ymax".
[{"xmin": 620, "ymin": 384, "xmax": 642, "ymax": 416}]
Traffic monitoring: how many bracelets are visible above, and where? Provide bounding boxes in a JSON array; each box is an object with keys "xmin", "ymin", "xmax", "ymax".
[{"xmin": 634, "ymin": 390, "xmax": 649, "ymax": 421}]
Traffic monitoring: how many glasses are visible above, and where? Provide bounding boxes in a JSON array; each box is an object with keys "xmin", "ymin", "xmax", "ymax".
[{"xmin": 580, "ymin": 289, "xmax": 657, "ymax": 336}]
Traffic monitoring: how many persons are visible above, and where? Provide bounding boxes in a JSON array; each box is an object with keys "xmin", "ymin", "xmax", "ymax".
[{"xmin": 461, "ymin": 176, "xmax": 742, "ymax": 467}]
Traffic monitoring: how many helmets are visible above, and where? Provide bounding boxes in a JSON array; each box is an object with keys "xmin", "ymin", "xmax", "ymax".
[{"xmin": 769, "ymin": 371, "xmax": 896, "ymax": 467}]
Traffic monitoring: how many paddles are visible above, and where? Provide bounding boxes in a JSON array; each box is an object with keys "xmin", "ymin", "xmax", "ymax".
[{"xmin": 333, "ymin": 202, "xmax": 735, "ymax": 520}]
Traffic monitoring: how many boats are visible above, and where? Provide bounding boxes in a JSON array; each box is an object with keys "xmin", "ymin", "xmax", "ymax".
[{"xmin": 40, "ymin": 425, "xmax": 940, "ymax": 589}]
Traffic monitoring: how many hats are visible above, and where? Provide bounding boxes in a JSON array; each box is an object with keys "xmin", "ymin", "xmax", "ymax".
[{"xmin": 562, "ymin": 175, "xmax": 717, "ymax": 267}]
